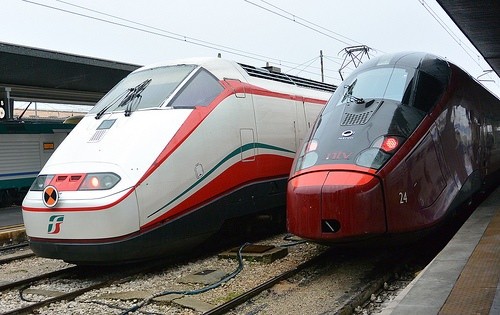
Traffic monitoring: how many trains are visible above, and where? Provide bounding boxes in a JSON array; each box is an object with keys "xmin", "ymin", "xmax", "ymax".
[{"xmin": 0, "ymin": 52, "xmax": 500, "ymax": 268}]
